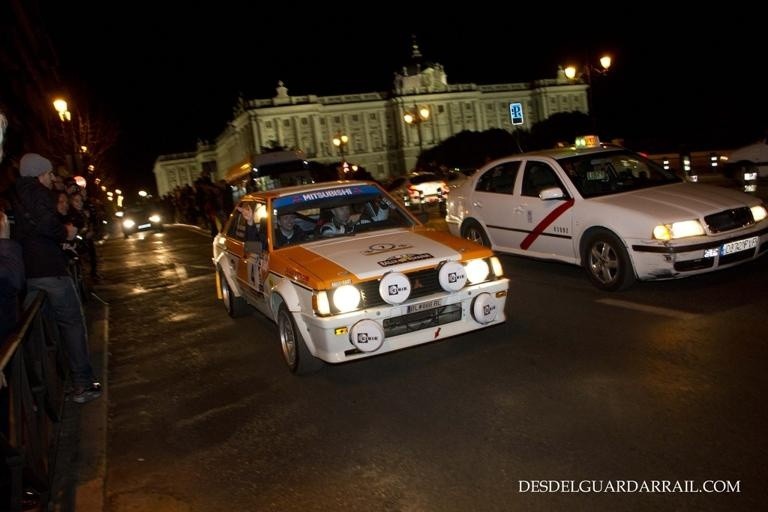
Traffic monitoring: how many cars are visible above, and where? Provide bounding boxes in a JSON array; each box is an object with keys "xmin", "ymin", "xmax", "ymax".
[{"xmin": 121, "ymin": 202, "xmax": 164, "ymax": 238}]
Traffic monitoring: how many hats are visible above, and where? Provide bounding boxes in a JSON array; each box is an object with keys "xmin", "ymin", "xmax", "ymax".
[{"xmin": 19, "ymin": 153, "xmax": 53, "ymax": 177}]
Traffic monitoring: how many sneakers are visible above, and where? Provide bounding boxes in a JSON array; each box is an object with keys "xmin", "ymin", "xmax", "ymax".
[{"xmin": 72, "ymin": 383, "xmax": 103, "ymax": 404}]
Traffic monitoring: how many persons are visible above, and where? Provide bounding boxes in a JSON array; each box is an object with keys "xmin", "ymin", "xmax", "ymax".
[
  {"xmin": 317, "ymin": 197, "xmax": 391, "ymax": 237},
  {"xmin": 234, "ymin": 201, "xmax": 305, "ymax": 249},
  {"xmin": 0, "ymin": 108, "xmax": 115, "ymax": 512},
  {"xmin": 135, "ymin": 171, "xmax": 242, "ymax": 239}
]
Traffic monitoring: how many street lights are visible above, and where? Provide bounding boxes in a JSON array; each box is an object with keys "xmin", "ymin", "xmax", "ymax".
[
  {"xmin": 403, "ymin": 108, "xmax": 430, "ymax": 154},
  {"xmin": 564, "ymin": 54, "xmax": 614, "ymax": 109},
  {"xmin": 331, "ymin": 129, "xmax": 349, "ymax": 160},
  {"xmin": 53, "ymin": 98, "xmax": 125, "ymax": 209}
]
